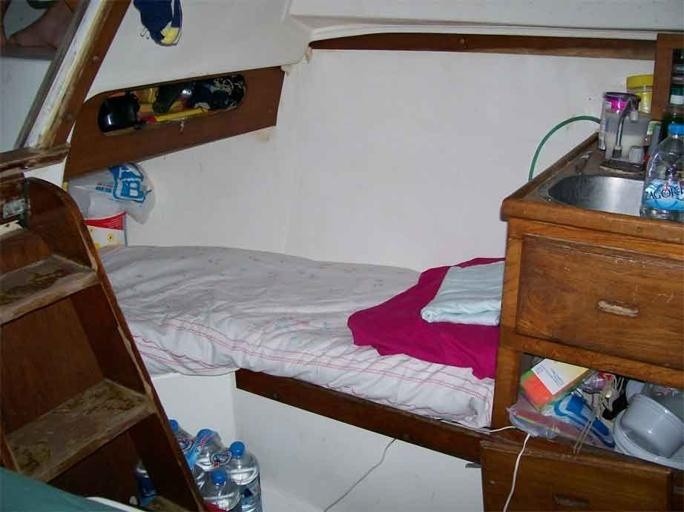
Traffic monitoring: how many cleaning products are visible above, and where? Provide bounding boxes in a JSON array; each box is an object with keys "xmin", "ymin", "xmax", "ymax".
[{"xmin": 639, "ymin": 122, "xmax": 683, "ymax": 223}]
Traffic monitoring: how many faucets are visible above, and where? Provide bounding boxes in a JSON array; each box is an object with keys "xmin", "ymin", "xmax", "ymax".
[{"xmin": 612, "ymin": 95, "xmax": 640, "ymax": 156}]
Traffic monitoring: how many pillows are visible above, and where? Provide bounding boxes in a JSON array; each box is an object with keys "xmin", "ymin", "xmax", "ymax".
[{"xmin": 349, "ymin": 256, "xmax": 494, "ymax": 378}]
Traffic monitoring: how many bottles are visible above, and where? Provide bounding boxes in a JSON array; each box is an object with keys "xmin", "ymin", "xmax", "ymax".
[
  {"xmin": 640, "ymin": 95, "xmax": 684, "ymax": 224},
  {"xmin": 167, "ymin": 418, "xmax": 262, "ymax": 512},
  {"xmin": 626, "ymin": 73, "xmax": 654, "ymax": 114}
]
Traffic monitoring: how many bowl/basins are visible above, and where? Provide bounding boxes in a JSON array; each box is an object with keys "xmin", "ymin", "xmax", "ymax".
[{"xmin": 621, "ymin": 394, "xmax": 684, "ymax": 458}]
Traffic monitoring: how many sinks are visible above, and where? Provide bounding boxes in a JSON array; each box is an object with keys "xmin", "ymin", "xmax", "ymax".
[{"xmin": 540, "ymin": 170, "xmax": 644, "ymax": 217}]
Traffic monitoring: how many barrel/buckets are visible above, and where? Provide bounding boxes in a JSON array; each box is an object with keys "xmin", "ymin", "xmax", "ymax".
[{"xmin": 614, "ymin": 408, "xmax": 683, "ymax": 468}]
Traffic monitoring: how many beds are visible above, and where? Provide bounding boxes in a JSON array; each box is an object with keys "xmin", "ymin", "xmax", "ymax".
[{"xmin": 85, "ymin": 241, "xmax": 511, "ymax": 466}]
